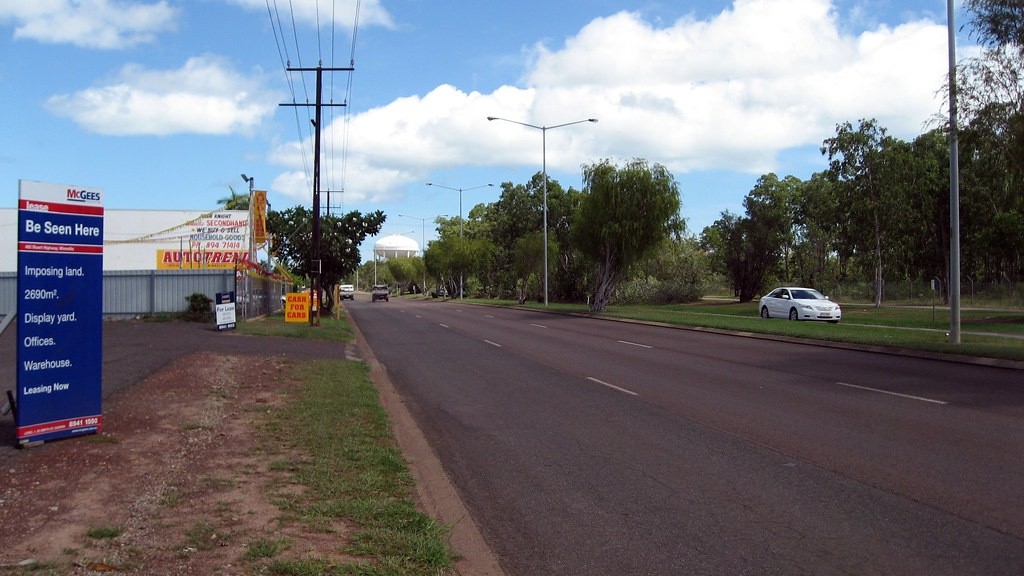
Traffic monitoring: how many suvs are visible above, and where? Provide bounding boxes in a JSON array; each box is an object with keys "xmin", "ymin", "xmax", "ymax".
[{"xmin": 371, "ymin": 284, "xmax": 390, "ymax": 302}]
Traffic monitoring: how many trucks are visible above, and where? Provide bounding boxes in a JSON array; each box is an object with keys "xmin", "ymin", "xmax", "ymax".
[{"xmin": 339, "ymin": 284, "xmax": 355, "ymax": 301}]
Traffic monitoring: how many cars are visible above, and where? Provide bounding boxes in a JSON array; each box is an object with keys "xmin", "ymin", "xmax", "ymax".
[
  {"xmin": 439, "ymin": 287, "xmax": 448, "ymax": 297},
  {"xmin": 237, "ymin": 287, "xmax": 286, "ymax": 310},
  {"xmin": 758, "ymin": 286, "xmax": 841, "ymax": 324}
]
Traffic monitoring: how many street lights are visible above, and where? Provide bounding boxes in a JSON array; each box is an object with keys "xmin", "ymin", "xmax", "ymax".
[
  {"xmin": 487, "ymin": 116, "xmax": 598, "ymax": 305},
  {"xmin": 399, "ymin": 214, "xmax": 448, "ymax": 255},
  {"xmin": 426, "ymin": 182, "xmax": 495, "ymax": 299}
]
